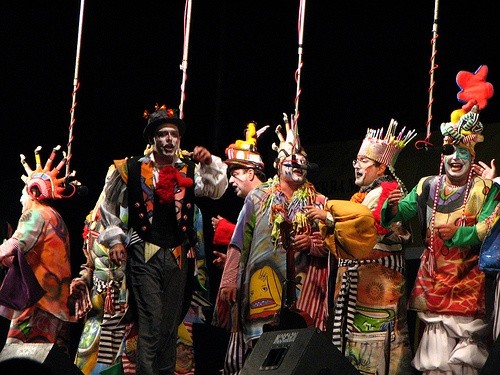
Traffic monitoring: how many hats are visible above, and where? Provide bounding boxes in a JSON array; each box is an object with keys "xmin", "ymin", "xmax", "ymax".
[
  {"xmin": 270, "ymin": 113, "xmax": 307, "ymax": 165},
  {"xmin": 440, "ymin": 65, "xmax": 494, "ymax": 145},
  {"xmin": 223, "ymin": 123, "xmax": 270, "ymax": 171},
  {"xmin": 143, "ymin": 104, "xmax": 182, "ymax": 137},
  {"xmin": 358, "ymin": 120, "xmax": 416, "ymax": 165},
  {"xmin": 19, "ymin": 145, "xmax": 80, "ymax": 201}
]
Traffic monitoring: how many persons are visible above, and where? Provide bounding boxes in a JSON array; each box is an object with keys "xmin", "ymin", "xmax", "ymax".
[
  {"xmin": 303, "ymin": 118, "xmax": 415, "ymax": 375},
  {"xmin": 212, "ymin": 114, "xmax": 330, "ymax": 375},
  {"xmin": 0, "ymin": 146, "xmax": 81, "ymax": 365},
  {"xmin": 69, "ymin": 104, "xmax": 266, "ymax": 375},
  {"xmin": 380, "ymin": 64, "xmax": 500, "ymax": 375}
]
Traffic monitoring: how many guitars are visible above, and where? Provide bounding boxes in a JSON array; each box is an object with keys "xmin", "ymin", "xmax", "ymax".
[{"xmin": 274, "ymin": 219, "xmax": 314, "ymax": 330}]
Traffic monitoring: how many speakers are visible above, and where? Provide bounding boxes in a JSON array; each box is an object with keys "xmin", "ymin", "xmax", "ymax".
[
  {"xmin": 0, "ymin": 342, "xmax": 83, "ymax": 375},
  {"xmin": 238, "ymin": 327, "xmax": 361, "ymax": 375}
]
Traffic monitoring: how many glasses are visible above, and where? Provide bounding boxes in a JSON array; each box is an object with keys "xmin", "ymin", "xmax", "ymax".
[{"xmin": 353, "ymin": 160, "xmax": 370, "ymax": 167}]
